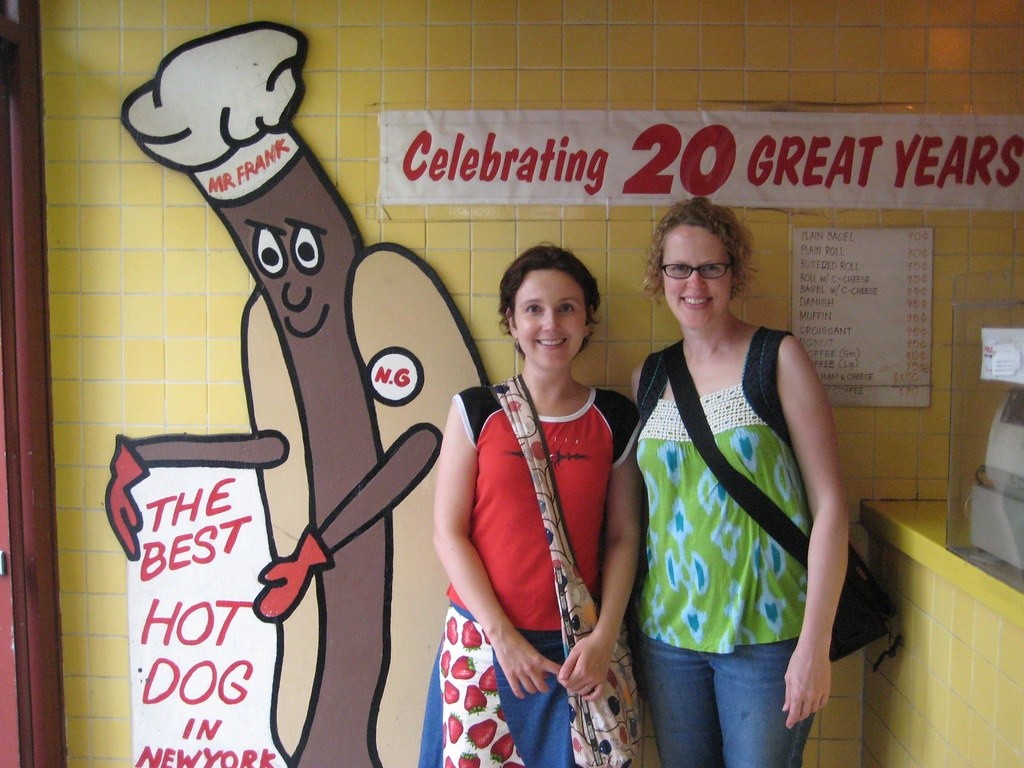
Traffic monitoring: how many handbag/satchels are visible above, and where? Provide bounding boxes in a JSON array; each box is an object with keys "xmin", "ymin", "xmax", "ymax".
[
  {"xmin": 562, "ymin": 619, "xmax": 643, "ymax": 768},
  {"xmin": 829, "ymin": 541, "xmax": 903, "ymax": 674}
]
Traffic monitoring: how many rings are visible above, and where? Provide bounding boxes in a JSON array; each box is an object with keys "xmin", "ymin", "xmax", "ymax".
[{"xmin": 585, "ymin": 685, "xmax": 591, "ymax": 691}]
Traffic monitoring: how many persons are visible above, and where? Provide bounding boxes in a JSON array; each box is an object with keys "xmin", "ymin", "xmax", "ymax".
[
  {"xmin": 419, "ymin": 245, "xmax": 644, "ymax": 767},
  {"xmin": 632, "ymin": 197, "xmax": 849, "ymax": 768}
]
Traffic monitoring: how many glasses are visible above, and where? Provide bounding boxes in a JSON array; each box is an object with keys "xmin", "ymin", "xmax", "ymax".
[{"xmin": 659, "ymin": 260, "xmax": 732, "ymax": 279}]
